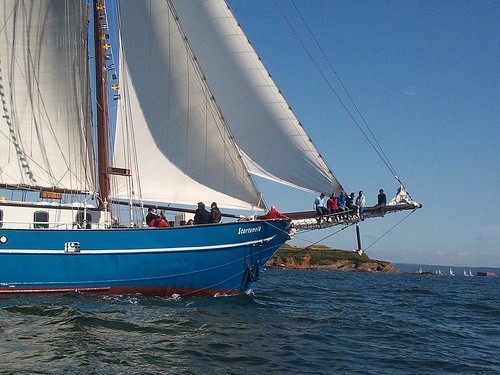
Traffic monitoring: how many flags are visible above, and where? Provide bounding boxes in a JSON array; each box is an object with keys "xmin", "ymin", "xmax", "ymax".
[{"xmin": 95, "ymin": 4, "xmax": 121, "ymax": 101}]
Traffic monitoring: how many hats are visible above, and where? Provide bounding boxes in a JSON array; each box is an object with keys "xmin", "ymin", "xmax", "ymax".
[
  {"xmin": 211, "ymin": 202, "xmax": 216, "ymax": 208},
  {"xmin": 341, "ymin": 192, "xmax": 345, "ymax": 196}
]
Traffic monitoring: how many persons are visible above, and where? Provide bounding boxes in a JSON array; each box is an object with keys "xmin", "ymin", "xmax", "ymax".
[
  {"xmin": 312, "ymin": 192, "xmax": 330, "ymax": 224},
  {"xmin": 373, "ymin": 189, "xmax": 387, "ymax": 218},
  {"xmin": 327, "ymin": 193, "xmax": 342, "ymax": 222},
  {"xmin": 265, "ymin": 205, "xmax": 288, "ymax": 219},
  {"xmin": 355, "ymin": 191, "xmax": 365, "ymax": 221},
  {"xmin": 346, "ymin": 193, "xmax": 358, "ymax": 219},
  {"xmin": 146, "ymin": 208, "xmax": 170, "ymax": 228},
  {"xmin": 209, "ymin": 201, "xmax": 222, "ymax": 224},
  {"xmin": 193, "ymin": 202, "xmax": 211, "ymax": 224},
  {"xmin": 337, "ymin": 192, "xmax": 351, "ymax": 221},
  {"xmin": 187, "ymin": 219, "xmax": 194, "ymax": 225}
]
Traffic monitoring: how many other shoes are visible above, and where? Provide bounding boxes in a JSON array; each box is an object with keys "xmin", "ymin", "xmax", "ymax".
[{"xmin": 319, "ymin": 214, "xmax": 364, "ymax": 224}]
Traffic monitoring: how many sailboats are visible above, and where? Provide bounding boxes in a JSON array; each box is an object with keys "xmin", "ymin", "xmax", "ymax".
[
  {"xmin": 416, "ymin": 266, "xmax": 475, "ymax": 278},
  {"xmin": 0, "ymin": 0, "xmax": 423, "ymax": 298}
]
